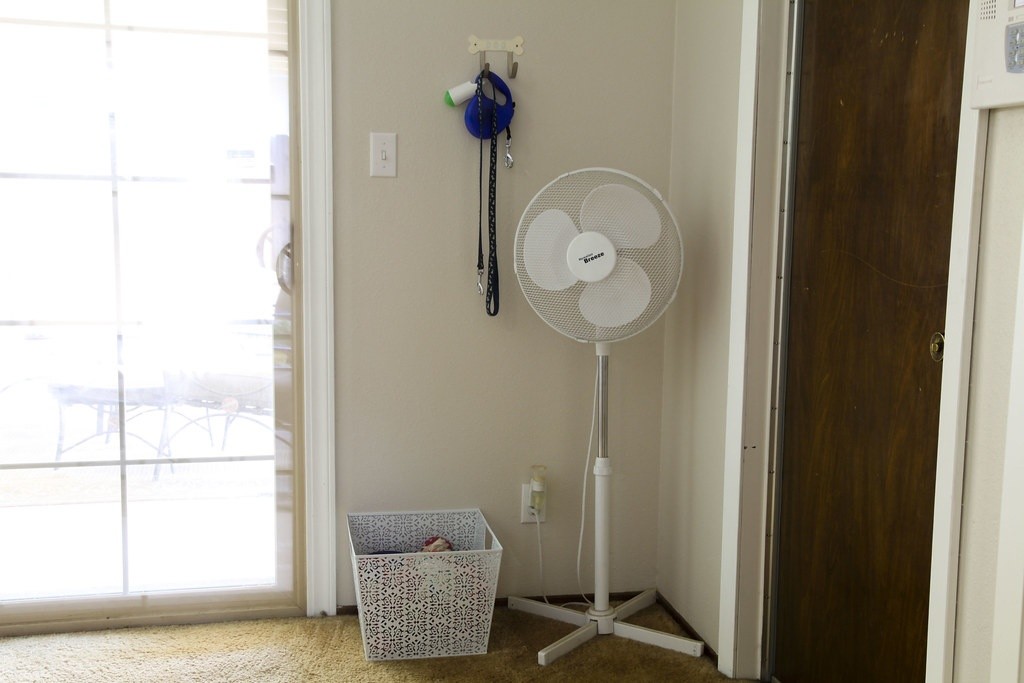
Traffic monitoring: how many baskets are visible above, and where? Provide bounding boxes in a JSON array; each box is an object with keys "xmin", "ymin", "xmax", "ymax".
[{"xmin": 347, "ymin": 498, "xmax": 503, "ymax": 662}]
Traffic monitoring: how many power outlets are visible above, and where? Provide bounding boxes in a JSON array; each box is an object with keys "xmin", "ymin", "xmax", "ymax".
[{"xmin": 520, "ymin": 481, "xmax": 546, "ymax": 524}]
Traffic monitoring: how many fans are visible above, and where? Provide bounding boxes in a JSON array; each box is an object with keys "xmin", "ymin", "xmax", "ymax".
[{"xmin": 507, "ymin": 164, "xmax": 705, "ymax": 668}]
